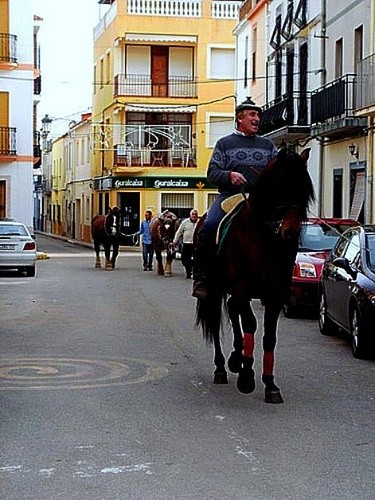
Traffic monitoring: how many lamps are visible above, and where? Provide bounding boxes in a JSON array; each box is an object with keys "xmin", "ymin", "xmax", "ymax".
[
  {"xmin": 89, "ymin": 183, "xmax": 93, "ymax": 190},
  {"xmin": 34, "ymin": 114, "xmax": 52, "ymax": 139},
  {"xmin": 349, "ymin": 142, "xmax": 359, "ymax": 159}
]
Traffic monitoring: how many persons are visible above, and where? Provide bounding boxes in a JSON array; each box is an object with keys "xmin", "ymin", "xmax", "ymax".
[
  {"xmin": 192, "ymin": 104, "xmax": 281, "ymax": 298},
  {"xmin": 172, "ymin": 209, "xmax": 200, "ymax": 279},
  {"xmin": 132, "ymin": 211, "xmax": 154, "ymax": 271}
]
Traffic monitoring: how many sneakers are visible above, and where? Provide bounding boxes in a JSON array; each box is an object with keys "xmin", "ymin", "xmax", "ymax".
[{"xmin": 193, "ymin": 280, "xmax": 208, "ymax": 299}]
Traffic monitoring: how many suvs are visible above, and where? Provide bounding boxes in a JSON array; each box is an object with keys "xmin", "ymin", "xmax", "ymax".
[{"xmin": 283, "ymin": 217, "xmax": 362, "ymax": 318}]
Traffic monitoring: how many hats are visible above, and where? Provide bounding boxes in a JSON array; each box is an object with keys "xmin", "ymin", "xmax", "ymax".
[{"xmin": 235, "ymin": 102, "xmax": 261, "ymax": 112}]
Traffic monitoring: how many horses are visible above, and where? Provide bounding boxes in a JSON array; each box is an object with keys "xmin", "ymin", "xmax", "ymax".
[
  {"xmin": 149, "ymin": 212, "xmax": 179, "ymax": 277},
  {"xmin": 90, "ymin": 206, "xmax": 123, "ymax": 270},
  {"xmin": 192, "ymin": 145, "xmax": 317, "ymax": 405}
]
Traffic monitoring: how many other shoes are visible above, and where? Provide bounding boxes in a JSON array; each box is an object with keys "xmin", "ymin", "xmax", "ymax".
[
  {"xmin": 149, "ymin": 267, "xmax": 152, "ymax": 271},
  {"xmin": 144, "ymin": 267, "xmax": 147, "ymax": 271},
  {"xmin": 186, "ymin": 273, "xmax": 192, "ymax": 279}
]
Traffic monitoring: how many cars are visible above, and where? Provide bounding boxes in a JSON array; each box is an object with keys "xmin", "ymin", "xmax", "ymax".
[
  {"xmin": 0, "ymin": 219, "xmax": 37, "ymax": 277},
  {"xmin": 317, "ymin": 224, "xmax": 375, "ymax": 359}
]
